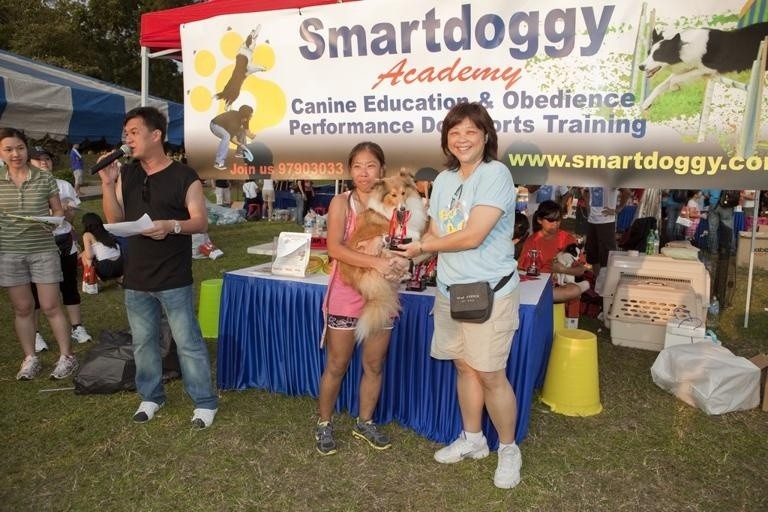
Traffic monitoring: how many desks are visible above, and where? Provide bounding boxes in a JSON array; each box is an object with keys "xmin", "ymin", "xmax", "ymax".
[
  {"xmin": 304, "ymin": 192, "xmax": 333, "ymax": 210},
  {"xmin": 246, "ymin": 187, "xmax": 295, "ymax": 210},
  {"xmin": 213, "ymin": 253, "xmax": 552, "ymax": 451}
]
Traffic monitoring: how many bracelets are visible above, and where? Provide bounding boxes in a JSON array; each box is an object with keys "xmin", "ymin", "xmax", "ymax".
[
  {"xmin": 408, "ymin": 259, "xmax": 414, "ymax": 273},
  {"xmin": 419, "ymin": 241, "xmax": 424, "ymax": 255}
]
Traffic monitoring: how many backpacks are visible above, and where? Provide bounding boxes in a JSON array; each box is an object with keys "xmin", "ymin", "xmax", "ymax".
[{"xmin": 719, "ymin": 190, "xmax": 740, "ymax": 208}]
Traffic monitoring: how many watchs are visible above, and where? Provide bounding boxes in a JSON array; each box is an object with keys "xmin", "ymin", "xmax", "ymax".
[{"xmin": 169, "ymin": 219, "xmax": 182, "ymax": 235}]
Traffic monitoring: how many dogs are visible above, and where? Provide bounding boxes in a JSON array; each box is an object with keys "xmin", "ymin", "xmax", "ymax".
[
  {"xmin": 638, "ymin": 21, "xmax": 768, "ymax": 111},
  {"xmin": 338, "ymin": 165, "xmax": 428, "ymax": 350},
  {"xmin": 211, "ymin": 29, "xmax": 267, "ymax": 112},
  {"xmin": 552, "ymin": 243, "xmax": 581, "ymax": 287}
]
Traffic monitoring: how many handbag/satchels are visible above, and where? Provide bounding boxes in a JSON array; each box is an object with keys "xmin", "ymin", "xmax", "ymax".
[
  {"xmin": 199, "ymin": 241, "xmax": 224, "ymax": 261},
  {"xmin": 663, "ymin": 317, "xmax": 706, "ymax": 348},
  {"xmin": 676, "ymin": 206, "xmax": 699, "ymax": 228},
  {"xmin": 81, "ymin": 264, "xmax": 99, "ymax": 295},
  {"xmin": 450, "ymin": 281, "xmax": 494, "ymax": 323}
]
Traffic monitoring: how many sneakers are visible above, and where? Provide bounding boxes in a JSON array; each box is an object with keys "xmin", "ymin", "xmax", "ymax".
[
  {"xmin": 433, "ymin": 429, "xmax": 490, "ymax": 464},
  {"xmin": 351, "ymin": 416, "xmax": 392, "ymax": 451},
  {"xmin": 16, "ymin": 355, "xmax": 42, "ymax": 380},
  {"xmin": 315, "ymin": 416, "xmax": 338, "ymax": 456},
  {"xmin": 70, "ymin": 326, "xmax": 92, "ymax": 344},
  {"xmin": 191, "ymin": 407, "xmax": 218, "ymax": 430},
  {"xmin": 50, "ymin": 355, "xmax": 80, "ymax": 380},
  {"xmin": 213, "ymin": 163, "xmax": 227, "ymax": 170},
  {"xmin": 493, "ymin": 445, "xmax": 523, "ymax": 489},
  {"xmin": 35, "ymin": 333, "xmax": 49, "ymax": 352},
  {"xmin": 132, "ymin": 400, "xmax": 166, "ymax": 424},
  {"xmin": 233, "ymin": 152, "xmax": 243, "ymax": 158}
]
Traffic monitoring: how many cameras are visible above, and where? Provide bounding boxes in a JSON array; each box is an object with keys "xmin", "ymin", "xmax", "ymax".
[{"xmin": 390, "ymin": 237, "xmax": 412, "ymax": 252}]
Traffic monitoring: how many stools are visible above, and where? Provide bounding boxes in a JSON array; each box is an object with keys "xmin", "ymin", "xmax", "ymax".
[
  {"xmin": 549, "ymin": 302, "xmax": 565, "ymax": 336},
  {"xmin": 246, "ymin": 203, "xmax": 260, "ymax": 218},
  {"xmin": 314, "ymin": 207, "xmax": 327, "ymax": 216}
]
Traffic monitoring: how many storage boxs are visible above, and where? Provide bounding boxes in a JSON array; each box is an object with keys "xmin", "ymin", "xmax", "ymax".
[
  {"xmin": 733, "ymin": 231, "xmax": 767, "ymax": 273},
  {"xmin": 746, "ymin": 351, "xmax": 767, "ymax": 415}
]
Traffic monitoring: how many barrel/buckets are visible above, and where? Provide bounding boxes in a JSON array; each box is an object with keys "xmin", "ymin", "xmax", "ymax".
[{"xmin": 552, "ymin": 303, "xmax": 566, "ymax": 336}]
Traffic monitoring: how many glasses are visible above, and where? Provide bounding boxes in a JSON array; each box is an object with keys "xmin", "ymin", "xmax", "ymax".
[
  {"xmin": 542, "ymin": 217, "xmax": 561, "ymax": 222},
  {"xmin": 142, "ymin": 176, "xmax": 151, "ymax": 203}
]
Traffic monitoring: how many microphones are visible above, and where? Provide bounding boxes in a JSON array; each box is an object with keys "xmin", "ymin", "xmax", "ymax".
[{"xmin": 87, "ymin": 143, "xmax": 131, "ymax": 176}]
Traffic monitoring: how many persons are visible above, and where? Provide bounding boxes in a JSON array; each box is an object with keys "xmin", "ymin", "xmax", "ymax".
[
  {"xmin": 0, "ymin": 128, "xmax": 79, "ymax": 381},
  {"xmin": 312, "ymin": 141, "xmax": 397, "ymax": 455},
  {"xmin": 214, "ymin": 180, "xmax": 232, "ymax": 205},
  {"xmin": 242, "ymin": 180, "xmax": 315, "ymax": 225},
  {"xmin": 210, "ymin": 105, "xmax": 257, "ymax": 171},
  {"xmin": 81, "ymin": 213, "xmax": 124, "ymax": 294},
  {"xmin": 70, "ymin": 143, "xmax": 87, "ymax": 196},
  {"xmin": 382, "ymin": 103, "xmax": 522, "ymax": 489},
  {"xmin": 95, "ymin": 107, "xmax": 219, "ymax": 431},
  {"xmin": 27, "ymin": 145, "xmax": 93, "ymax": 353},
  {"xmin": 512, "ymin": 185, "xmax": 757, "ymax": 303}
]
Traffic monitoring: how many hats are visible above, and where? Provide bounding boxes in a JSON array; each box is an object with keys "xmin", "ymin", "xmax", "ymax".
[{"xmin": 30, "ymin": 146, "xmax": 55, "ymax": 160}]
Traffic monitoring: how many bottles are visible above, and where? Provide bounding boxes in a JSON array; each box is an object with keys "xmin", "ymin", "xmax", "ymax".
[
  {"xmin": 645, "ymin": 228, "xmax": 660, "ymax": 256},
  {"xmin": 710, "ymin": 295, "xmax": 719, "ymax": 317},
  {"xmin": 302, "ymin": 207, "xmax": 316, "ymax": 232}
]
